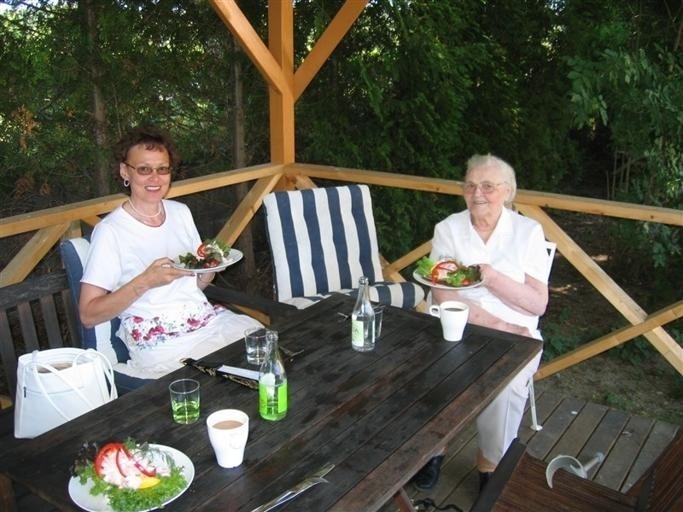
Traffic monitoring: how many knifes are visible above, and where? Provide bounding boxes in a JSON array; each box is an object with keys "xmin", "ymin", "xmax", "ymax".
[{"xmin": 248, "ymin": 463, "xmax": 337, "ymax": 512}]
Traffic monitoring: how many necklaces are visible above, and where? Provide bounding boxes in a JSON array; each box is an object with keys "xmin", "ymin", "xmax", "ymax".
[{"xmin": 127, "ymin": 195, "xmax": 163, "ymax": 217}]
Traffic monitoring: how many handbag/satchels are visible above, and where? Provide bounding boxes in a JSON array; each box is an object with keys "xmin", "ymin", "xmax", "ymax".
[{"xmin": 14, "ymin": 347, "xmax": 119, "ymax": 439}]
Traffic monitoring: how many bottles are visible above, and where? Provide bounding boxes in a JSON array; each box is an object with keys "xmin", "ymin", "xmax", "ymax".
[
  {"xmin": 258, "ymin": 331, "xmax": 289, "ymax": 420},
  {"xmin": 351, "ymin": 277, "xmax": 376, "ymax": 351}
]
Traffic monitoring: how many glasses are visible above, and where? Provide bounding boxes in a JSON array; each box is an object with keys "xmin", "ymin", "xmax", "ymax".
[
  {"xmin": 124, "ymin": 161, "xmax": 173, "ymax": 175},
  {"xmin": 460, "ymin": 181, "xmax": 510, "ymax": 194}
]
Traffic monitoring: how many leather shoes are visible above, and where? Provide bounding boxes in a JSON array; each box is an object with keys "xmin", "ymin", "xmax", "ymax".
[
  {"xmin": 478, "ymin": 470, "xmax": 494, "ymax": 494},
  {"xmin": 413, "ymin": 455, "xmax": 446, "ymax": 493}
]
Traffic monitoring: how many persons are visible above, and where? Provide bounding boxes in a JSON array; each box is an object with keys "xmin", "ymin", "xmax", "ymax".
[
  {"xmin": 409, "ymin": 148, "xmax": 554, "ymax": 502},
  {"xmin": 74, "ymin": 121, "xmax": 271, "ymax": 379}
]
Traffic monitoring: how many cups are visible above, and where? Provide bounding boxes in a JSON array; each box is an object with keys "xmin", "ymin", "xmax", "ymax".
[
  {"xmin": 430, "ymin": 301, "xmax": 470, "ymax": 342},
  {"xmin": 169, "ymin": 379, "xmax": 202, "ymax": 423},
  {"xmin": 244, "ymin": 328, "xmax": 268, "ymax": 364},
  {"xmin": 205, "ymin": 409, "xmax": 248, "ymax": 468},
  {"xmin": 373, "ymin": 306, "xmax": 384, "ymax": 338}
]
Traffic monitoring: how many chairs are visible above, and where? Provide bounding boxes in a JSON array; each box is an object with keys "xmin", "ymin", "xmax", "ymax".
[{"xmin": 467, "ymin": 425, "xmax": 683, "ymax": 510}]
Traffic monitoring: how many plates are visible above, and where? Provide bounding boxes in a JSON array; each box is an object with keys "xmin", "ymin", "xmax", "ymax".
[
  {"xmin": 413, "ymin": 264, "xmax": 486, "ymax": 289},
  {"xmin": 172, "ymin": 248, "xmax": 243, "ymax": 272},
  {"xmin": 68, "ymin": 442, "xmax": 195, "ymax": 512}
]
